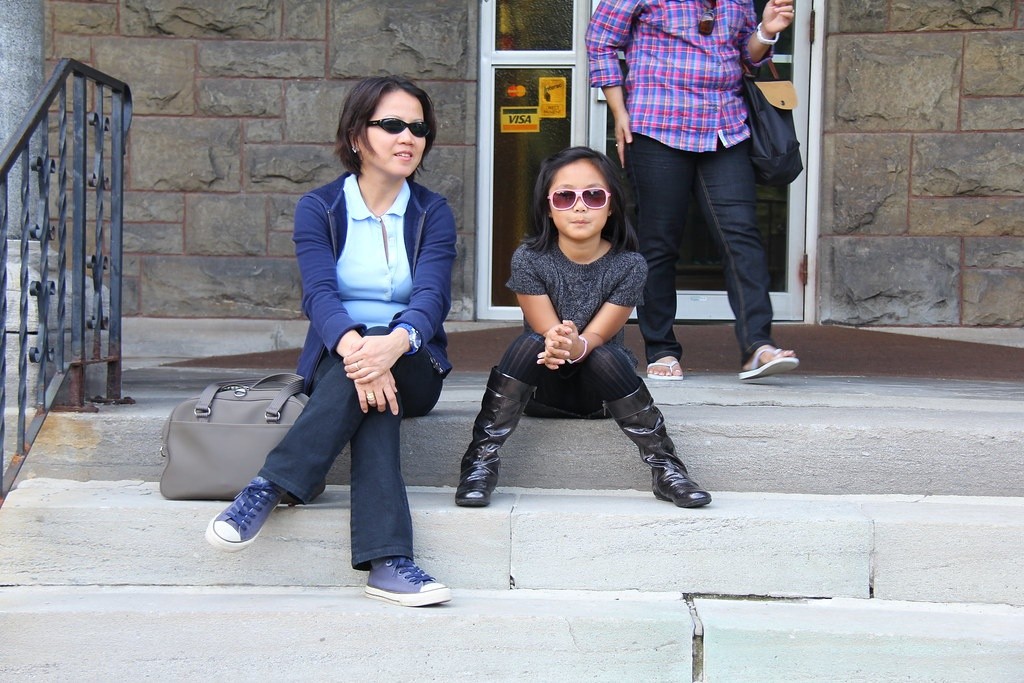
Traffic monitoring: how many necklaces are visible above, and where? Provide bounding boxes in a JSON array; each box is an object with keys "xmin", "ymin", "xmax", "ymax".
[{"xmin": 363, "ymin": 198, "xmax": 390, "ymax": 222}]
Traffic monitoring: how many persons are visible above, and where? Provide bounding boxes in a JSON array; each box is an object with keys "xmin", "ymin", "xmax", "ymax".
[
  {"xmin": 205, "ymin": 75, "xmax": 458, "ymax": 607},
  {"xmin": 455, "ymin": 146, "xmax": 713, "ymax": 507},
  {"xmin": 585, "ymin": 0, "xmax": 798, "ymax": 380}
]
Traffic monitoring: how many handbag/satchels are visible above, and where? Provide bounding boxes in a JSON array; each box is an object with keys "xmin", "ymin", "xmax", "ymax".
[
  {"xmin": 159, "ymin": 373, "xmax": 326, "ymax": 505},
  {"xmin": 741, "ymin": 30, "xmax": 803, "ymax": 187}
]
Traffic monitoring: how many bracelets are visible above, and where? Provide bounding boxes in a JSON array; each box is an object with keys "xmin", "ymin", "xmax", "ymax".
[{"xmin": 757, "ymin": 22, "xmax": 779, "ymax": 44}]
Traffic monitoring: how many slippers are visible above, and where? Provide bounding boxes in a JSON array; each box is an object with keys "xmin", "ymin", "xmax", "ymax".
[
  {"xmin": 738, "ymin": 348, "xmax": 799, "ymax": 380},
  {"xmin": 646, "ymin": 359, "xmax": 684, "ymax": 381}
]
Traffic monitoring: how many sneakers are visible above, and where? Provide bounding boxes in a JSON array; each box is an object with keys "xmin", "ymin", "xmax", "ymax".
[
  {"xmin": 363, "ymin": 557, "xmax": 452, "ymax": 606},
  {"xmin": 205, "ymin": 477, "xmax": 288, "ymax": 552}
]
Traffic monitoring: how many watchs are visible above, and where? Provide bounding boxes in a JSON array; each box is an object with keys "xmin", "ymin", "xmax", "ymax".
[
  {"xmin": 567, "ymin": 337, "xmax": 588, "ymax": 364},
  {"xmin": 392, "ymin": 323, "xmax": 422, "ymax": 355}
]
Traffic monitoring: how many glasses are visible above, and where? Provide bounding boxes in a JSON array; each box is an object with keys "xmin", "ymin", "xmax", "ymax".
[
  {"xmin": 366, "ymin": 117, "xmax": 431, "ymax": 138},
  {"xmin": 546, "ymin": 186, "xmax": 611, "ymax": 210},
  {"xmin": 698, "ymin": 0, "xmax": 715, "ymax": 36}
]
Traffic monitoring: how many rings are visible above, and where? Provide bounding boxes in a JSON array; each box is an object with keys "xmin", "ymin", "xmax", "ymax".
[
  {"xmin": 356, "ymin": 363, "xmax": 360, "ymax": 370},
  {"xmin": 792, "ymin": 9, "xmax": 794, "ymax": 12},
  {"xmin": 615, "ymin": 144, "xmax": 618, "ymax": 146},
  {"xmin": 367, "ymin": 393, "xmax": 375, "ymax": 402}
]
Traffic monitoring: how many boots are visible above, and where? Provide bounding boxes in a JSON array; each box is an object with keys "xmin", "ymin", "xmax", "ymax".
[
  {"xmin": 455, "ymin": 364, "xmax": 535, "ymax": 506},
  {"xmin": 602, "ymin": 375, "xmax": 711, "ymax": 507}
]
